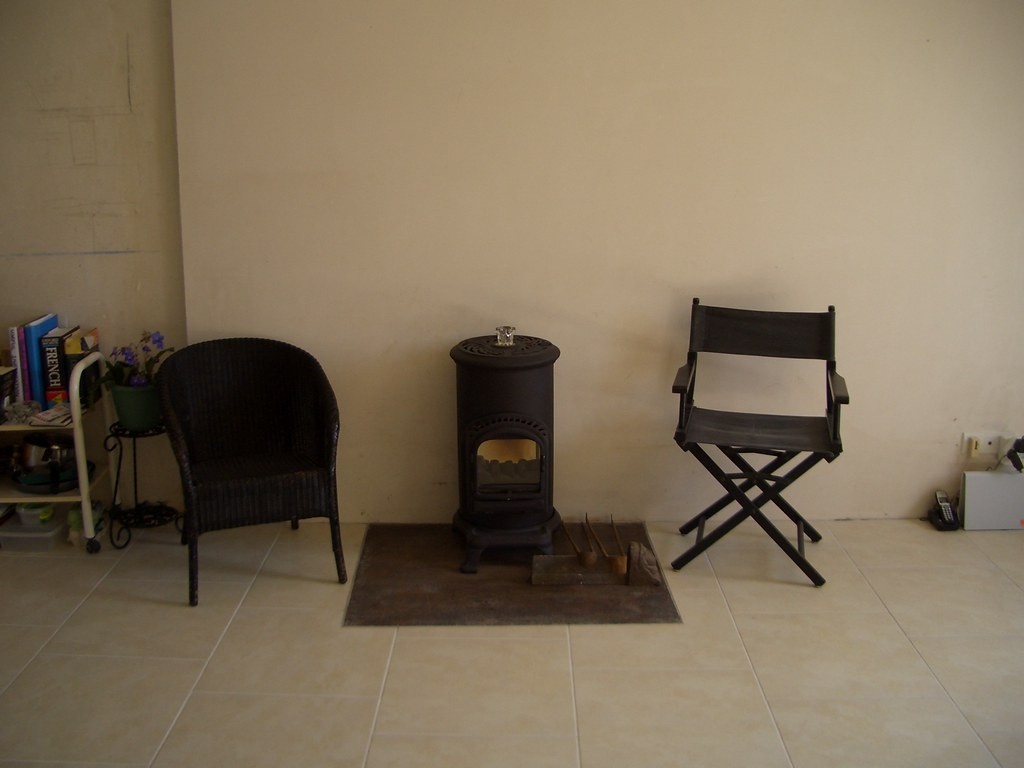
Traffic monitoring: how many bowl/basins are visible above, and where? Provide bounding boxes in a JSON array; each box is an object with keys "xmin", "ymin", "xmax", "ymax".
[
  {"xmin": 16, "ymin": 503, "xmax": 56, "ymax": 524},
  {"xmin": 9, "ymin": 432, "xmax": 75, "ymax": 492}
]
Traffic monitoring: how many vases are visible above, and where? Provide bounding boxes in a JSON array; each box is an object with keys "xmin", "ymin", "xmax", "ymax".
[{"xmin": 110, "ymin": 379, "xmax": 169, "ymax": 434}]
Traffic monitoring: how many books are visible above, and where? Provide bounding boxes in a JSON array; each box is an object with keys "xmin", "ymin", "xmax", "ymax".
[{"xmin": 0, "ymin": 313, "xmax": 100, "ymax": 426}]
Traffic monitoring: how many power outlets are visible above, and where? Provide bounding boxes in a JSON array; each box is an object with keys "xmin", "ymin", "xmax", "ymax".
[{"xmin": 959, "ymin": 430, "xmax": 1011, "ymax": 465}]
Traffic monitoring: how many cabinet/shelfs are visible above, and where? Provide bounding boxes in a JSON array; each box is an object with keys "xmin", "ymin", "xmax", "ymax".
[{"xmin": 0, "ymin": 348, "xmax": 124, "ymax": 556}]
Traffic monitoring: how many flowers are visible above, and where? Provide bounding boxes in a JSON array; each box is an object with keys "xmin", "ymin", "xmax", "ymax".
[{"xmin": 103, "ymin": 322, "xmax": 169, "ymax": 385}]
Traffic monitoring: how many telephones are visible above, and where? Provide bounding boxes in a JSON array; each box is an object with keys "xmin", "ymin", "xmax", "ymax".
[{"xmin": 927, "ymin": 490, "xmax": 960, "ymax": 531}]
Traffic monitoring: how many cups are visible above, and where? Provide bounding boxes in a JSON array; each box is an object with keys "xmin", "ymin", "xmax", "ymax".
[{"xmin": 496, "ymin": 326, "xmax": 515, "ymax": 345}]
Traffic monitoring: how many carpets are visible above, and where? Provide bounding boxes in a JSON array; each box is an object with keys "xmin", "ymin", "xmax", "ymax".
[{"xmin": 344, "ymin": 517, "xmax": 688, "ymax": 626}]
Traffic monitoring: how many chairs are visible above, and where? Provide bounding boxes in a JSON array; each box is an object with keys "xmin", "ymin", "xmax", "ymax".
[
  {"xmin": 670, "ymin": 297, "xmax": 851, "ymax": 589},
  {"xmin": 156, "ymin": 337, "xmax": 348, "ymax": 608}
]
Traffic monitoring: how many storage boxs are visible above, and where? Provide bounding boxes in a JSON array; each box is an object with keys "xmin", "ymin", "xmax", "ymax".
[{"xmin": 4, "ymin": 501, "xmax": 108, "ymax": 555}]
